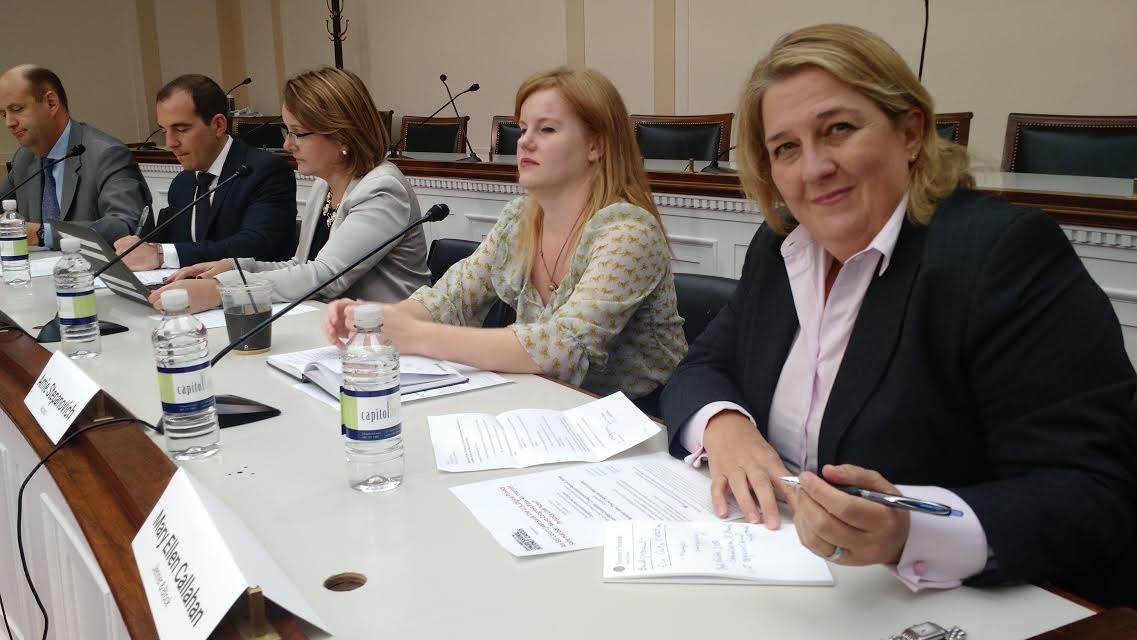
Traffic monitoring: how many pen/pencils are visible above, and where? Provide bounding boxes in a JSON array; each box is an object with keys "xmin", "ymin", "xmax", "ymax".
[
  {"xmin": 777, "ymin": 475, "xmax": 965, "ymax": 519},
  {"xmin": 134, "ymin": 206, "xmax": 150, "ymax": 237}
]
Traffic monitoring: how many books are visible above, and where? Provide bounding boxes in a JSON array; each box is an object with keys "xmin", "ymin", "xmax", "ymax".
[{"xmin": 265, "ymin": 344, "xmax": 468, "ymax": 404}]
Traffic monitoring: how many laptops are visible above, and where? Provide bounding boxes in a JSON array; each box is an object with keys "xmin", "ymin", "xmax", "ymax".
[{"xmin": 45, "ymin": 217, "xmax": 225, "ymax": 312}]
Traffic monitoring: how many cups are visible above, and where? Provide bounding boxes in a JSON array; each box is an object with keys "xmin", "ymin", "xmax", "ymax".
[{"xmin": 218, "ymin": 279, "xmax": 274, "ymax": 356}]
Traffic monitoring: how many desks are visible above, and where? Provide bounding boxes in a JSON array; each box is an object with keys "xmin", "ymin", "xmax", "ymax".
[
  {"xmin": 0, "ymin": 240, "xmax": 1135, "ymax": 638},
  {"xmin": 118, "ymin": 153, "xmax": 1137, "ymax": 400}
]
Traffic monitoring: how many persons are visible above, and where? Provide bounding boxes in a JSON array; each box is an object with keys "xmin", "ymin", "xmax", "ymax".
[
  {"xmin": 0, "ymin": 64, "xmax": 154, "ymax": 251},
  {"xmin": 148, "ymin": 68, "xmax": 431, "ymax": 314},
  {"xmin": 660, "ymin": 23, "xmax": 1135, "ymax": 608},
  {"xmin": 323, "ymin": 65, "xmax": 690, "ymax": 418},
  {"xmin": 114, "ymin": 75, "xmax": 298, "ymax": 272}
]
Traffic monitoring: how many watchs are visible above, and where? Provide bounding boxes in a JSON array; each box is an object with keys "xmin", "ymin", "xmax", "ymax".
[
  {"xmin": 36, "ymin": 223, "xmax": 44, "ymax": 247},
  {"xmin": 157, "ymin": 244, "xmax": 164, "ymax": 268}
]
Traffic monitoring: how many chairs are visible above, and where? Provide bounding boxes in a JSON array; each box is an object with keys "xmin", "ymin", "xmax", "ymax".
[
  {"xmin": 228, "ymin": 109, "xmax": 1137, "ymax": 201},
  {"xmin": 422, "ymin": 232, "xmax": 767, "ymax": 417}
]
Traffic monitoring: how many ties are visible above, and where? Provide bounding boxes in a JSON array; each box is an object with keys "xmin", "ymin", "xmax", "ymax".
[
  {"xmin": 195, "ymin": 173, "xmax": 216, "ymax": 243},
  {"xmin": 41, "ymin": 158, "xmax": 60, "ymax": 223}
]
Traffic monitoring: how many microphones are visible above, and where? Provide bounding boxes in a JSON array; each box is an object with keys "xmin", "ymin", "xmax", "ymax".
[
  {"xmin": 35, "ymin": 161, "xmax": 253, "ymax": 343},
  {"xmin": 387, "ymin": 83, "xmax": 479, "ymax": 158},
  {"xmin": 225, "ymin": 78, "xmax": 252, "ymax": 98},
  {"xmin": 157, "ymin": 204, "xmax": 450, "ymax": 434},
  {"xmin": 0, "ymin": 144, "xmax": 87, "ymax": 204},
  {"xmin": 440, "ymin": 74, "xmax": 482, "ymax": 162}
]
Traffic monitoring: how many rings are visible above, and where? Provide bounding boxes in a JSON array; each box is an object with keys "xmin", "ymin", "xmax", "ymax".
[{"xmin": 826, "ymin": 546, "xmax": 843, "ymax": 561}]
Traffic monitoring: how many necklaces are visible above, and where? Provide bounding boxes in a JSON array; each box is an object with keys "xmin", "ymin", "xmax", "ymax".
[
  {"xmin": 323, "ymin": 188, "xmax": 341, "ymax": 228},
  {"xmin": 540, "ymin": 206, "xmax": 585, "ymax": 292}
]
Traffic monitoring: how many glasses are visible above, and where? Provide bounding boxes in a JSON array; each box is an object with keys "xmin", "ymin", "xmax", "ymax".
[{"xmin": 279, "ymin": 126, "xmax": 317, "ymax": 145}]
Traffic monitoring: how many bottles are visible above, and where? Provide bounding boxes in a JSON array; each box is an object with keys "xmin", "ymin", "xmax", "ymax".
[
  {"xmin": 51, "ymin": 233, "xmax": 103, "ymax": 362},
  {"xmin": 0, "ymin": 198, "xmax": 31, "ymax": 287},
  {"xmin": 341, "ymin": 303, "xmax": 403, "ymax": 497},
  {"xmin": 152, "ymin": 287, "xmax": 222, "ymax": 460}
]
